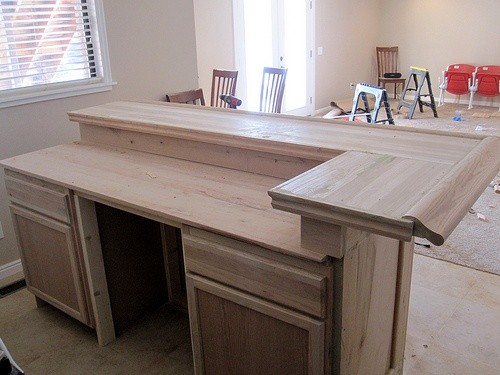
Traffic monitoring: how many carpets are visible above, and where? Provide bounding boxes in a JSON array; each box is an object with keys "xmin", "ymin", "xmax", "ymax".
[{"xmin": 323, "ymin": 103, "xmax": 500, "ymax": 276}]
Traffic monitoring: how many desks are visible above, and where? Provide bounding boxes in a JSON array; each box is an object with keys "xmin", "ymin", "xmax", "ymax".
[{"xmin": 0, "ymin": 143, "xmax": 415, "ymax": 375}]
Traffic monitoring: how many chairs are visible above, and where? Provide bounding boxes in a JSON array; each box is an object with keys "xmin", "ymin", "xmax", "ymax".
[
  {"xmin": 438, "ymin": 64, "xmax": 500, "ymax": 110},
  {"xmin": 210, "ymin": 67, "xmax": 238, "ymax": 109},
  {"xmin": 166, "ymin": 88, "xmax": 207, "ymax": 106},
  {"xmin": 259, "ymin": 67, "xmax": 288, "ymax": 114},
  {"xmin": 376, "ymin": 46, "xmax": 406, "ymax": 99}
]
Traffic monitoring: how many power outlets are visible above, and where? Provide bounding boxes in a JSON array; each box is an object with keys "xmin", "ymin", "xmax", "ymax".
[{"xmin": 350, "ymin": 83, "xmax": 355, "ymax": 89}]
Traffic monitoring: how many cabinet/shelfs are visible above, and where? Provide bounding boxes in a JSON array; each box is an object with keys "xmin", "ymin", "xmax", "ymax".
[
  {"xmin": 191, "ymin": 225, "xmax": 351, "ymax": 375},
  {"xmin": 6, "ymin": 168, "xmax": 92, "ymax": 335}
]
